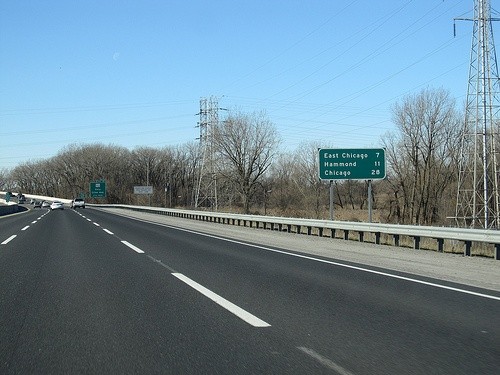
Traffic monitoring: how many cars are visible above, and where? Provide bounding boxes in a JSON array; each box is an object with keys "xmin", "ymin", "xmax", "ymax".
[
  {"xmin": 42, "ymin": 201, "xmax": 50, "ymax": 207},
  {"xmin": 29, "ymin": 198, "xmax": 36, "ymax": 205},
  {"xmin": 70, "ymin": 201, "xmax": 74, "ymax": 208},
  {"xmin": 18, "ymin": 200, "xmax": 24, "ymax": 204},
  {"xmin": 50, "ymin": 201, "xmax": 64, "ymax": 210},
  {"xmin": 34, "ymin": 201, "xmax": 41, "ymax": 208}
]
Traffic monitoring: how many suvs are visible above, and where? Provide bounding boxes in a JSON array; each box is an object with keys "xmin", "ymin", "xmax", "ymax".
[{"xmin": 73, "ymin": 199, "xmax": 85, "ymax": 209}]
